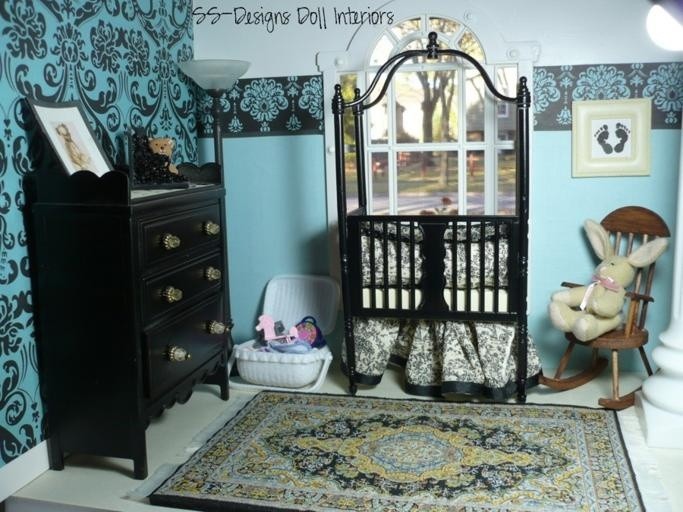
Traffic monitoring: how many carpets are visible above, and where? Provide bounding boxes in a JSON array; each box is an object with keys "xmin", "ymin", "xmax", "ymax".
[{"xmin": 148, "ymin": 388, "xmax": 647, "ymax": 512}]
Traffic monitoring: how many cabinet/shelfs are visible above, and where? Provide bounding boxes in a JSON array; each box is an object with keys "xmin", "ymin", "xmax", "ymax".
[{"xmin": 19, "ymin": 162, "xmax": 237, "ymax": 480}]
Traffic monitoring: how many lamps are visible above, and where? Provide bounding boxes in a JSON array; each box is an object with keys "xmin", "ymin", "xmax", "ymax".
[
  {"xmin": 645, "ymin": 0, "xmax": 683, "ymax": 51},
  {"xmin": 174, "ymin": 58, "xmax": 248, "ymax": 182}
]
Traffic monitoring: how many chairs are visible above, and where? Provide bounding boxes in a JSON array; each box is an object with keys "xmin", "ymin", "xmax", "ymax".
[{"xmin": 536, "ymin": 205, "xmax": 672, "ymax": 411}]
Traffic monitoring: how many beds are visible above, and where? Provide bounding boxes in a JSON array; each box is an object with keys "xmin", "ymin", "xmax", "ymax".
[{"xmin": 331, "ymin": 28, "xmax": 532, "ymax": 405}]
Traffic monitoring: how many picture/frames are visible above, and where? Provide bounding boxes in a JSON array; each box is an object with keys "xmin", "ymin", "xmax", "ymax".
[
  {"xmin": 24, "ymin": 94, "xmax": 114, "ymax": 179},
  {"xmin": 570, "ymin": 98, "xmax": 653, "ymax": 179}
]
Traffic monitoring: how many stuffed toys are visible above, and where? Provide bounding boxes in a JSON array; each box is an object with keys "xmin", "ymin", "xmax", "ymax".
[
  {"xmin": 241, "ymin": 313, "xmax": 327, "ymax": 355},
  {"xmin": 547, "ymin": 218, "xmax": 669, "ymax": 342},
  {"xmin": 146, "ymin": 135, "xmax": 180, "ymax": 176}
]
